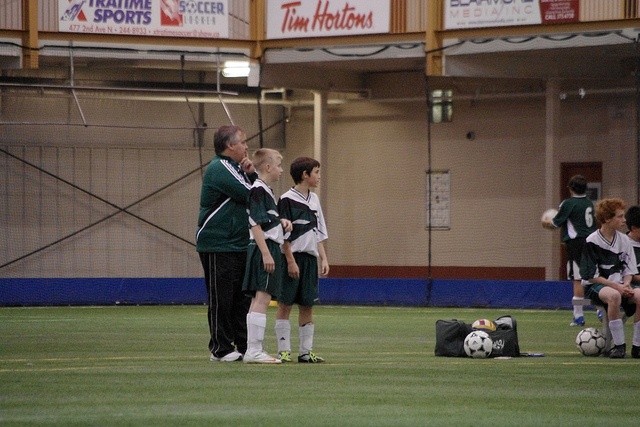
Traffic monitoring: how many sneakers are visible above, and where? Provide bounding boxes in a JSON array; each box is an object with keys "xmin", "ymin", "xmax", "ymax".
[
  {"xmin": 569, "ymin": 316, "xmax": 585, "ymax": 326},
  {"xmin": 632, "ymin": 345, "xmax": 640, "ymax": 358},
  {"xmin": 609, "ymin": 343, "xmax": 626, "ymax": 358},
  {"xmin": 298, "ymin": 352, "xmax": 325, "ymax": 363},
  {"xmin": 597, "ymin": 309, "xmax": 603, "ymax": 321},
  {"xmin": 210, "ymin": 348, "xmax": 243, "ymax": 362},
  {"xmin": 243, "ymin": 351, "xmax": 282, "ymax": 364},
  {"xmin": 277, "ymin": 351, "xmax": 292, "ymax": 362}
]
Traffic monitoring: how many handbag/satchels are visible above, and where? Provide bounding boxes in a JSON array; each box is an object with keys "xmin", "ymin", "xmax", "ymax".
[{"xmin": 435, "ymin": 315, "xmax": 520, "ymax": 359}]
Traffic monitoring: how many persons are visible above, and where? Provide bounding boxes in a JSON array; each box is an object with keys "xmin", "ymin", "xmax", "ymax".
[
  {"xmin": 194, "ymin": 124, "xmax": 259, "ymax": 363},
  {"xmin": 240, "ymin": 147, "xmax": 293, "ymax": 364},
  {"xmin": 276, "ymin": 156, "xmax": 330, "ymax": 364},
  {"xmin": 544, "ymin": 173, "xmax": 601, "ymax": 327},
  {"xmin": 578, "ymin": 196, "xmax": 639, "ymax": 360},
  {"xmin": 621, "ymin": 204, "xmax": 640, "ymax": 317}
]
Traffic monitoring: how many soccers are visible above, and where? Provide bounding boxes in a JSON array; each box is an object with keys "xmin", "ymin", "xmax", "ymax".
[
  {"xmin": 472, "ymin": 318, "xmax": 497, "ymax": 331},
  {"xmin": 463, "ymin": 330, "xmax": 493, "ymax": 358},
  {"xmin": 576, "ymin": 328, "xmax": 608, "ymax": 357},
  {"xmin": 542, "ymin": 209, "xmax": 559, "ymax": 228}
]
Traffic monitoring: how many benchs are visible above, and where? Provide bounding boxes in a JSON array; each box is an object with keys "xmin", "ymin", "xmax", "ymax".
[{"xmin": 570, "ymin": 296, "xmax": 640, "ymax": 354}]
